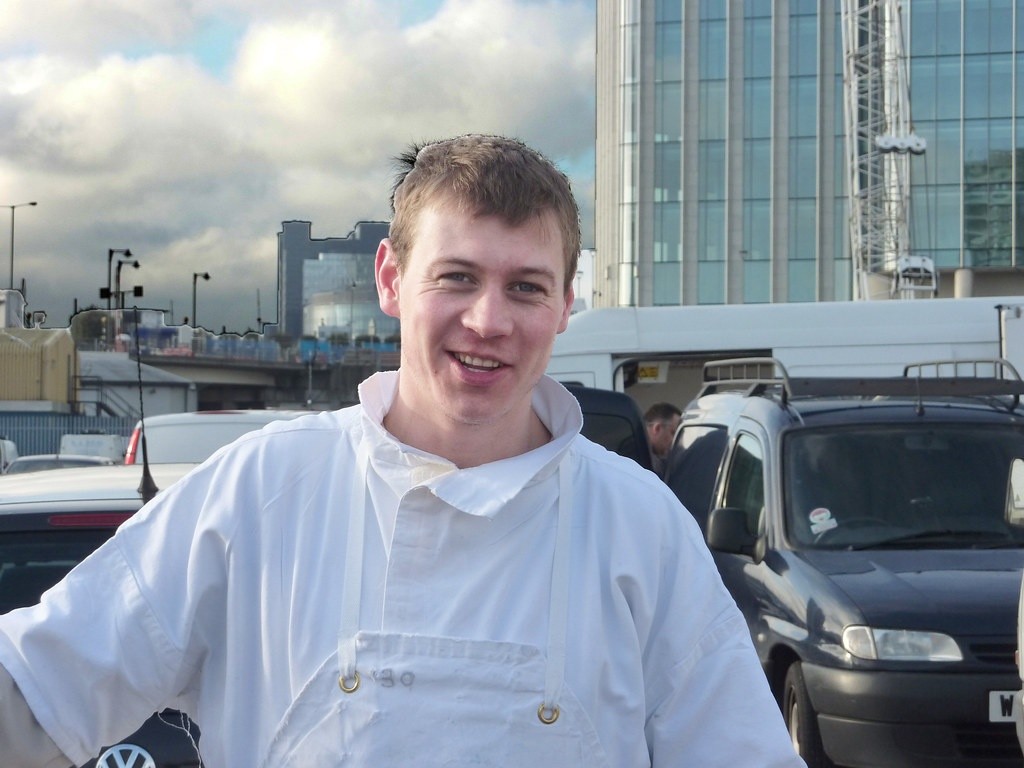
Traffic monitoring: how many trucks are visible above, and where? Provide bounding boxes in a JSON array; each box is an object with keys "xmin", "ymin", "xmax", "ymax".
[{"xmin": 544, "ymin": 297, "xmax": 1024, "ymax": 523}]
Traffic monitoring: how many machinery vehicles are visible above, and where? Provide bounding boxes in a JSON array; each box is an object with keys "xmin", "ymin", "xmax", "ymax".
[{"xmin": 842, "ymin": 0, "xmax": 938, "ymax": 302}]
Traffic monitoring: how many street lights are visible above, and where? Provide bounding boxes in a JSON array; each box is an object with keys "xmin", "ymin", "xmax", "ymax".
[
  {"xmin": 107, "ymin": 248, "xmax": 140, "ymax": 310},
  {"xmin": 995, "ymin": 303, "xmax": 1022, "ymax": 380},
  {"xmin": 192, "ymin": 271, "xmax": 211, "ymax": 329},
  {"xmin": 0, "ymin": 202, "xmax": 38, "ymax": 290}
]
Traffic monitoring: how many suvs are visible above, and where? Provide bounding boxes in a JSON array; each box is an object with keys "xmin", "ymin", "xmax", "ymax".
[{"xmin": 558, "ymin": 360, "xmax": 1024, "ymax": 768}]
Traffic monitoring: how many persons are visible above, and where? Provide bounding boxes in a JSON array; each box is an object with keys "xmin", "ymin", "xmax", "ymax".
[
  {"xmin": 0, "ymin": 133, "xmax": 807, "ymax": 768},
  {"xmin": 642, "ymin": 402, "xmax": 682, "ymax": 479}
]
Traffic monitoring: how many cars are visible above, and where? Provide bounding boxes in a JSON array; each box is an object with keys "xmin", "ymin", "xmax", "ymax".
[{"xmin": 0, "ymin": 409, "xmax": 318, "ymax": 616}]
